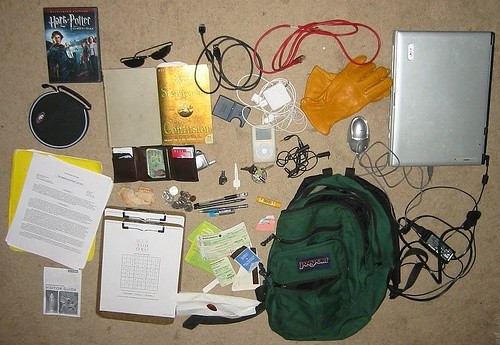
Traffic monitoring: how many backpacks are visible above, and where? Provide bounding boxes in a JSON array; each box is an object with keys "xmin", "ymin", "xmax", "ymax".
[{"xmin": 255, "ymin": 167, "xmax": 402, "ymax": 341}]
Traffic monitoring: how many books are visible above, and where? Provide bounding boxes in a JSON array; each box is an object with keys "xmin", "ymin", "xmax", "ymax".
[{"xmin": 101, "ymin": 64, "xmax": 214, "ymax": 147}]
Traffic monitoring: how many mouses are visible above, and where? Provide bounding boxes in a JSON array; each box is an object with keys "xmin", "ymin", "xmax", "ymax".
[{"xmin": 348, "ymin": 116, "xmax": 369, "ymax": 154}]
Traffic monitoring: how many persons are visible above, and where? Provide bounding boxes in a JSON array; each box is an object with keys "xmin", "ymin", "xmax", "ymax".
[
  {"xmin": 80, "ymin": 39, "xmax": 89, "ymax": 80},
  {"xmin": 59, "ymin": 297, "xmax": 78, "ymax": 314},
  {"xmin": 33, "ymin": 112, "xmax": 46, "ymax": 124},
  {"xmin": 148, "ymin": 169, "xmax": 165, "ymax": 178},
  {"xmin": 47, "ymin": 31, "xmax": 76, "ymax": 82},
  {"xmin": 87, "ymin": 36, "xmax": 99, "ymax": 81}
]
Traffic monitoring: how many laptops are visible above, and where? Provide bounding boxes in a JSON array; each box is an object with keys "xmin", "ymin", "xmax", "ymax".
[{"xmin": 388, "ymin": 31, "xmax": 494, "ymax": 166}]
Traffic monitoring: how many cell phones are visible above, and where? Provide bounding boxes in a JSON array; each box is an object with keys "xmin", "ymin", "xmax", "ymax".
[{"xmin": 195, "ymin": 149, "xmax": 216, "ymax": 171}]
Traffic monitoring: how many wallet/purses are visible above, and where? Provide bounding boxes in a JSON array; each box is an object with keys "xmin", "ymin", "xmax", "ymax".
[{"xmin": 111, "ymin": 143, "xmax": 198, "ymax": 182}]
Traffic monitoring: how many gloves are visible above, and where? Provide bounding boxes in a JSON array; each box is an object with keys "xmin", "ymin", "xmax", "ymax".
[
  {"xmin": 120, "ymin": 42, "xmax": 173, "ymax": 67},
  {"xmin": 300, "ymin": 54, "xmax": 393, "ymax": 136}
]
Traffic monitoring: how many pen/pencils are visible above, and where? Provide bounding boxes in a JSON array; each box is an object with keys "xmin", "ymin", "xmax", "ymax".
[
  {"xmin": 198, "ymin": 205, "xmax": 248, "ymax": 213},
  {"xmin": 209, "ymin": 192, "xmax": 248, "ymax": 204},
  {"xmin": 194, "ymin": 198, "xmax": 246, "ymax": 209}
]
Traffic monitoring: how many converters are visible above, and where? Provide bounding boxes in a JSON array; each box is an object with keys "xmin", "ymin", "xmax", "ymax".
[
  {"xmin": 263, "ymin": 82, "xmax": 292, "ymax": 112},
  {"xmin": 417, "ymin": 229, "xmax": 456, "ymax": 264}
]
